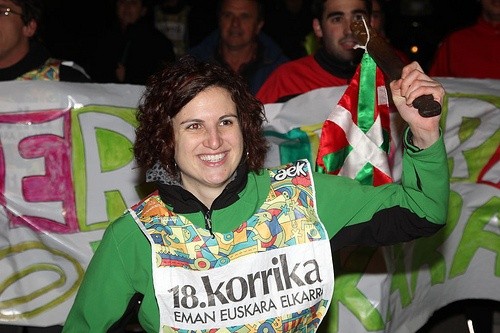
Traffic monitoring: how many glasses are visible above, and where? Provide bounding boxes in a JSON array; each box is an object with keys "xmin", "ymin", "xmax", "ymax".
[{"xmin": 0, "ymin": 7, "xmax": 22, "ymax": 16}]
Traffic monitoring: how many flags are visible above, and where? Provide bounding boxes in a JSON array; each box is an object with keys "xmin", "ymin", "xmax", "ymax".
[{"xmin": 313, "ymin": 49, "xmax": 392, "ymax": 185}]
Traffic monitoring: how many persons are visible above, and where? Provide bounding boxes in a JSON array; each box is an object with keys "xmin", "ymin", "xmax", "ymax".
[
  {"xmin": 370, "ymin": 0, "xmax": 390, "ymax": 45},
  {"xmin": 100, "ymin": 0, "xmax": 175, "ymax": 84},
  {"xmin": 154, "ymin": 0, "xmax": 192, "ymax": 56},
  {"xmin": 62, "ymin": 59, "xmax": 450, "ymax": 333},
  {"xmin": 0, "ymin": 0, "xmax": 94, "ymax": 83},
  {"xmin": 254, "ymin": 0, "xmax": 411, "ymax": 106},
  {"xmin": 185, "ymin": 0, "xmax": 288, "ymax": 95},
  {"xmin": 427, "ymin": 0, "xmax": 500, "ymax": 333}
]
